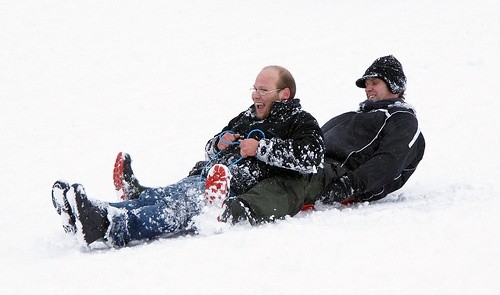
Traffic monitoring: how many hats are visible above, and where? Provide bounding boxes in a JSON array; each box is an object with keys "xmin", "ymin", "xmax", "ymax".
[{"xmin": 356, "ymin": 55, "xmax": 405, "ymax": 94}]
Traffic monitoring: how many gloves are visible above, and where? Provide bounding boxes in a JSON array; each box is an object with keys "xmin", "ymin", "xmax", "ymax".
[{"xmin": 317, "ymin": 176, "xmax": 354, "ymax": 205}]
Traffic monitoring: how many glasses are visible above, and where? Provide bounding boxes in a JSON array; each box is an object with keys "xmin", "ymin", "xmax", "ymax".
[{"xmin": 250, "ymin": 87, "xmax": 281, "ymax": 96}]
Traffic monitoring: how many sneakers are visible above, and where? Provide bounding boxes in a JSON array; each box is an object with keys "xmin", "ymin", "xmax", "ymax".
[
  {"xmin": 53, "ymin": 180, "xmax": 76, "ymax": 234},
  {"xmin": 205, "ymin": 164, "xmax": 230, "ymax": 223},
  {"xmin": 113, "ymin": 152, "xmax": 140, "ymax": 200},
  {"xmin": 72, "ymin": 183, "xmax": 107, "ymax": 246}
]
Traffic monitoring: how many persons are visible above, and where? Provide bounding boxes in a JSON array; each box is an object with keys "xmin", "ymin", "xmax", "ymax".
[
  {"xmin": 114, "ymin": 55, "xmax": 426, "ymax": 238},
  {"xmin": 51, "ymin": 65, "xmax": 325, "ymax": 250}
]
